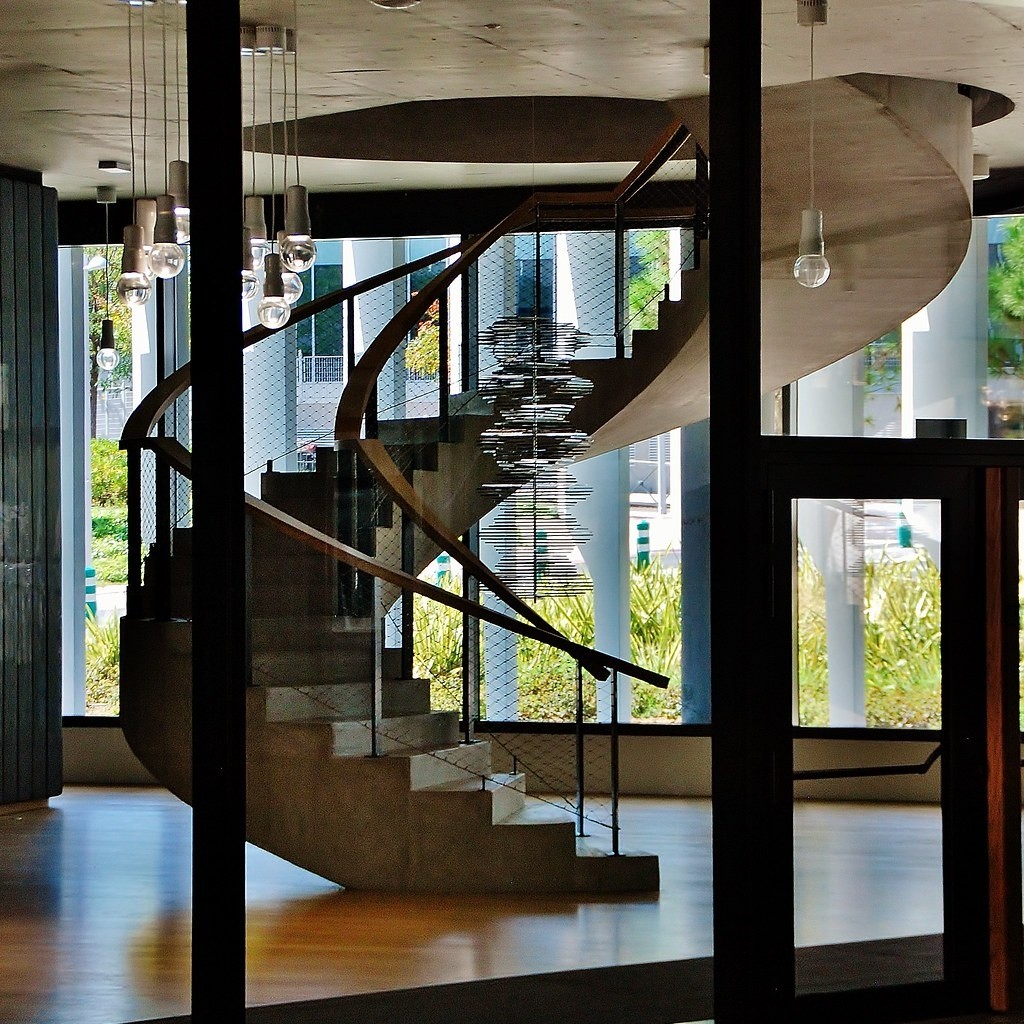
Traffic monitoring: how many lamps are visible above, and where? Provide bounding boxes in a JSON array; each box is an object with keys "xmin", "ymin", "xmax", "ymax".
[
  {"xmin": 791, "ymin": 0, "xmax": 829, "ymax": 288},
  {"xmin": 242, "ymin": 25, "xmax": 317, "ymax": 328},
  {"xmin": 95, "ymin": 0, "xmax": 190, "ymax": 371}
]
